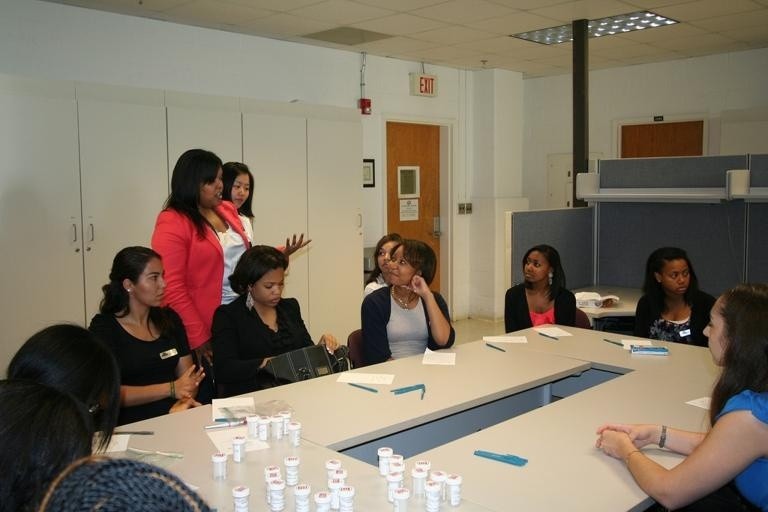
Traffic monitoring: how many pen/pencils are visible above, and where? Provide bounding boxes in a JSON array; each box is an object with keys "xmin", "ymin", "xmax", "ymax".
[
  {"xmin": 392, "ymin": 384, "xmax": 424, "ymax": 395},
  {"xmin": 486, "ymin": 343, "xmax": 506, "ymax": 352},
  {"xmin": 538, "ymin": 333, "xmax": 559, "ymax": 340},
  {"xmin": 215, "ymin": 418, "xmax": 246, "ymax": 420},
  {"xmin": 112, "ymin": 431, "xmax": 154, "ymax": 435},
  {"xmin": 348, "ymin": 382, "xmax": 377, "ymax": 393},
  {"xmin": 204, "ymin": 420, "xmax": 247, "ymax": 429},
  {"xmin": 477, "ymin": 451, "xmax": 528, "ymax": 464},
  {"xmin": 474, "ymin": 450, "xmax": 528, "ymax": 466},
  {"xmin": 604, "ymin": 339, "xmax": 624, "ymax": 346}
]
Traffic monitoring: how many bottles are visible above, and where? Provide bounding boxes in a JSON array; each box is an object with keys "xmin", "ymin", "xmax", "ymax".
[{"xmin": 210, "ymin": 410, "xmax": 462, "ymax": 512}]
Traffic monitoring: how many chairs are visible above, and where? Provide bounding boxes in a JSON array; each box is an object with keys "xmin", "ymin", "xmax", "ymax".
[
  {"xmin": 346, "ymin": 329, "xmax": 366, "ymax": 367},
  {"xmin": 575, "ymin": 307, "xmax": 589, "ymax": 329}
]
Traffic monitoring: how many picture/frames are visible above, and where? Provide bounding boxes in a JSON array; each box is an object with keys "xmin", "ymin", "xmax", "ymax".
[{"xmin": 362, "ymin": 159, "xmax": 375, "ymax": 187}]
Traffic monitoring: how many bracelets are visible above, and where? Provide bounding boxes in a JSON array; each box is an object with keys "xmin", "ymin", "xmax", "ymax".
[
  {"xmin": 626, "ymin": 450, "xmax": 642, "ymax": 468},
  {"xmin": 658, "ymin": 426, "xmax": 667, "ymax": 450},
  {"xmin": 169, "ymin": 379, "xmax": 176, "ymax": 398}
]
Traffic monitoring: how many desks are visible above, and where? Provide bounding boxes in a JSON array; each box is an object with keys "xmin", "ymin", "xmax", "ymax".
[{"xmin": 566, "ymin": 286, "xmax": 643, "ymax": 320}]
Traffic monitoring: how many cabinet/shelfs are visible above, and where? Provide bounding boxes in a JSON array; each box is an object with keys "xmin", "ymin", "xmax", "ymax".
[
  {"xmin": 0, "ymin": 75, "xmax": 168, "ymax": 384},
  {"xmin": 164, "ymin": 89, "xmax": 308, "ymax": 342},
  {"xmin": 307, "ymin": 102, "xmax": 365, "ymax": 347}
]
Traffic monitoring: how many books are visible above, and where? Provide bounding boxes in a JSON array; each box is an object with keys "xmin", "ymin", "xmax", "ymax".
[{"xmin": 631, "ymin": 347, "xmax": 669, "ymax": 355}]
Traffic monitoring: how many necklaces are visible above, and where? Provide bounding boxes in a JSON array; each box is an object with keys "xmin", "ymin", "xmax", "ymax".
[{"xmin": 390, "ymin": 285, "xmax": 418, "ymax": 310}]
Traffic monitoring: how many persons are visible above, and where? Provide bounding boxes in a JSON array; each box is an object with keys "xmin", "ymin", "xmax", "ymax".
[
  {"xmin": 363, "ymin": 233, "xmax": 403, "ymax": 300},
  {"xmin": 361, "ymin": 238, "xmax": 456, "ymax": 365},
  {"xmin": 632, "ymin": 246, "xmax": 717, "ymax": 347},
  {"xmin": 150, "ymin": 148, "xmax": 312, "ymax": 379},
  {"xmin": 88, "ymin": 245, "xmax": 206, "ymax": 426},
  {"xmin": 0, "ymin": 376, "xmax": 94, "ymax": 511},
  {"xmin": 504, "ymin": 244, "xmax": 577, "ymax": 332},
  {"xmin": 7, "ymin": 323, "xmax": 122, "ymax": 454},
  {"xmin": 211, "ymin": 245, "xmax": 340, "ymax": 398},
  {"xmin": 595, "ymin": 282, "xmax": 768, "ymax": 512},
  {"xmin": 223, "ymin": 161, "xmax": 255, "ymax": 245}
]
